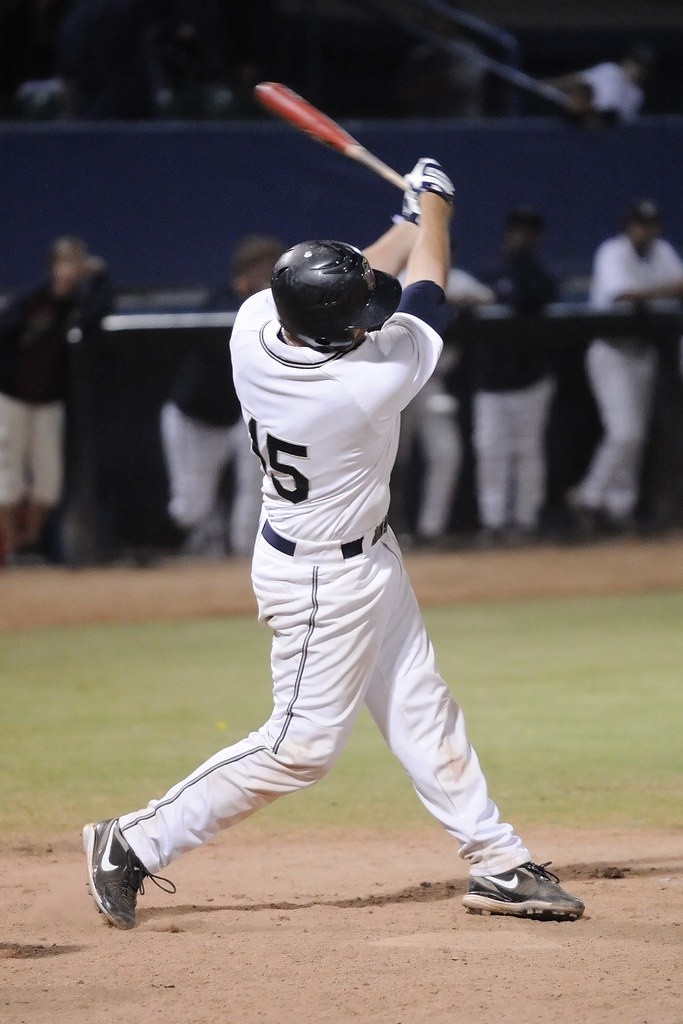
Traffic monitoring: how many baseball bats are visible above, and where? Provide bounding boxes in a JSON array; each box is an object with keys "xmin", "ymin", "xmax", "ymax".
[{"xmin": 253, "ymin": 81, "xmax": 411, "ymax": 192}]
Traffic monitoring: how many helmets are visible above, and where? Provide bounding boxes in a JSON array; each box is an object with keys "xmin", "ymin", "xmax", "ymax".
[{"xmin": 270, "ymin": 240, "xmax": 402, "ymax": 354}]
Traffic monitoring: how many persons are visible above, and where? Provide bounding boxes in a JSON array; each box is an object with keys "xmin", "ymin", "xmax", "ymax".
[
  {"xmin": 82, "ymin": 156, "xmax": 578, "ymax": 927},
  {"xmin": 0, "ymin": 45, "xmax": 683, "ymax": 570}
]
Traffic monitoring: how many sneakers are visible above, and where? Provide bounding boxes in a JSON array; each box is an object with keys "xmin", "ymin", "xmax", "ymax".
[
  {"xmin": 462, "ymin": 862, "xmax": 585, "ymax": 919},
  {"xmin": 80, "ymin": 818, "xmax": 176, "ymax": 929}
]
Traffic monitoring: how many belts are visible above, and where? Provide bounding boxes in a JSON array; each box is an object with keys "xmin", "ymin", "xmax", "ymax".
[{"xmin": 262, "ymin": 514, "xmax": 388, "ymax": 560}]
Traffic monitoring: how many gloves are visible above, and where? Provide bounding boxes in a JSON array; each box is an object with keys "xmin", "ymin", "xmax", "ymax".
[{"xmin": 394, "ymin": 157, "xmax": 456, "ymax": 229}]
